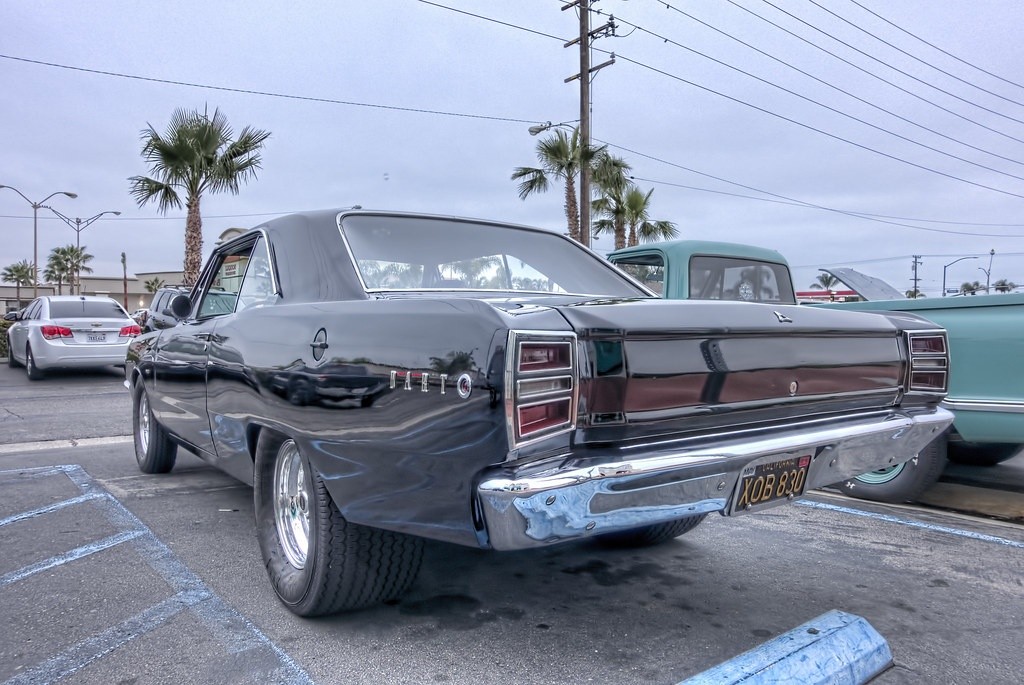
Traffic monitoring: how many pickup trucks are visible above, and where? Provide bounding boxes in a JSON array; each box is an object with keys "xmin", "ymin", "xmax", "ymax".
[{"xmin": 606, "ymin": 240, "xmax": 1024, "ymax": 506}]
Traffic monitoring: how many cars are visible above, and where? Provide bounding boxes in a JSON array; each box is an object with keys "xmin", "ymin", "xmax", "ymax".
[
  {"xmin": 3, "ymin": 295, "xmax": 144, "ymax": 381},
  {"xmin": 144, "ymin": 285, "xmax": 238, "ymax": 333},
  {"xmin": 124, "ymin": 204, "xmax": 954, "ymax": 618}
]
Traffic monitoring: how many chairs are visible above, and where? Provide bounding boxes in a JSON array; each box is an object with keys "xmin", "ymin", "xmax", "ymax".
[{"xmin": 433, "ymin": 279, "xmax": 467, "ymax": 288}]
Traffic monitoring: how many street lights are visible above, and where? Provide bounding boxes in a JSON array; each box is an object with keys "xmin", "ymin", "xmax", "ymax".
[
  {"xmin": 942, "ymin": 257, "xmax": 979, "ymax": 296},
  {"xmin": 30, "ymin": 204, "xmax": 122, "ymax": 295},
  {"xmin": 528, "ymin": 122, "xmax": 591, "ymax": 249},
  {"xmin": 978, "ymin": 247, "xmax": 996, "ymax": 294},
  {"xmin": 0, "ymin": 184, "xmax": 79, "ymax": 298}
]
